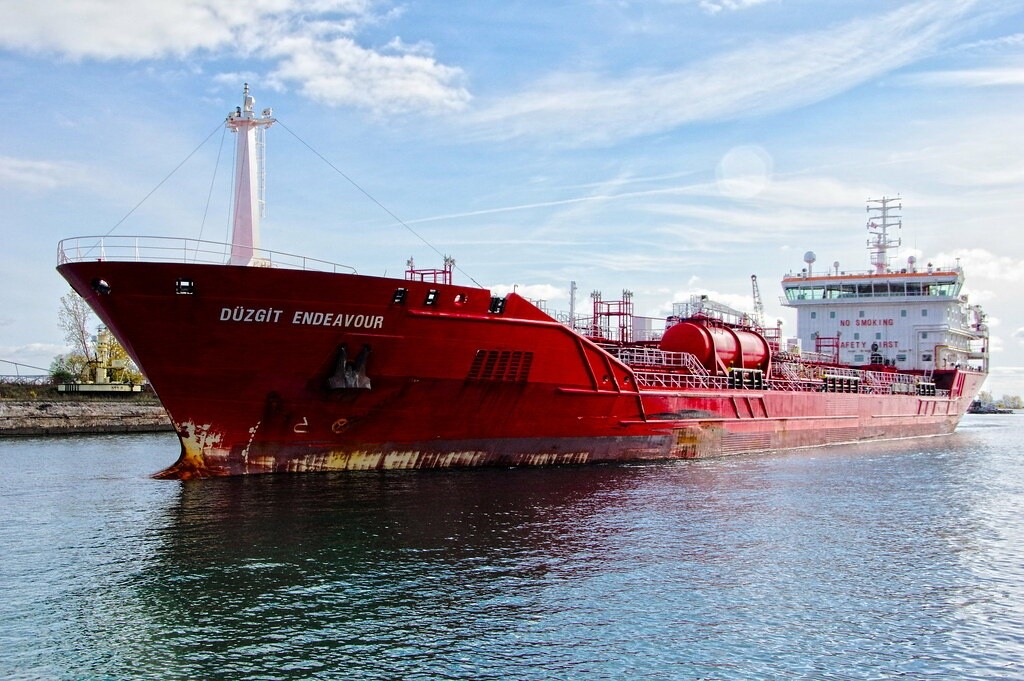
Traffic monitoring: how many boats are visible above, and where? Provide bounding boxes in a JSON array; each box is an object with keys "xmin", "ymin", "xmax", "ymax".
[
  {"xmin": 57, "ymin": 325, "xmax": 144, "ymax": 400},
  {"xmin": 966, "ymin": 399, "xmax": 1013, "ymax": 414},
  {"xmin": 55, "ymin": 82, "xmax": 991, "ymax": 484}
]
